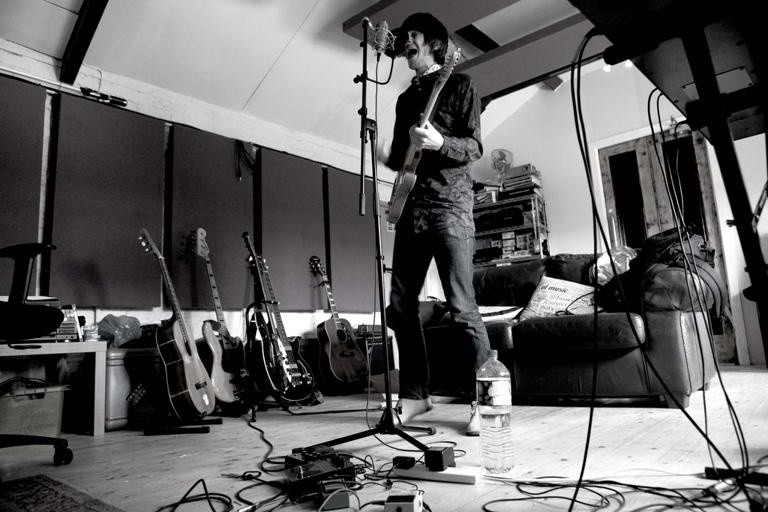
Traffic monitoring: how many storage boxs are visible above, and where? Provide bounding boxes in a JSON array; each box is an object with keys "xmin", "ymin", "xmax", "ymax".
[{"xmin": 0, "ymin": 383, "xmax": 70, "ymax": 447}]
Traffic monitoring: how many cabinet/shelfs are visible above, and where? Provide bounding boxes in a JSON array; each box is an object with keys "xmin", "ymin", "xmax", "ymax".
[
  {"xmin": 597, "ymin": 127, "xmax": 737, "ymax": 364},
  {"xmin": 517, "ymin": 274, "xmax": 604, "ymax": 323},
  {"xmin": 472, "ymin": 194, "xmax": 551, "ymax": 268}
]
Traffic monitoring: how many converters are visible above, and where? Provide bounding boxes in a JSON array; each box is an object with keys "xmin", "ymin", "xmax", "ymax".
[
  {"xmin": 427, "ymin": 447, "xmax": 454, "ymax": 470},
  {"xmin": 318, "ymin": 478, "xmax": 350, "ymax": 509},
  {"xmin": 282, "ymin": 456, "xmax": 356, "ymax": 503},
  {"xmin": 285, "ymin": 448, "xmax": 351, "ymax": 468},
  {"xmin": 385, "ymin": 489, "xmax": 423, "ymax": 512},
  {"xmin": 393, "ymin": 456, "xmax": 415, "ymax": 469}
]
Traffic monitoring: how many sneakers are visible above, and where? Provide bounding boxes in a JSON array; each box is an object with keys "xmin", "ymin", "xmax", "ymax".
[
  {"xmin": 392, "ymin": 396, "xmax": 434, "ymax": 426},
  {"xmin": 465, "ymin": 401, "xmax": 481, "ymax": 436}
]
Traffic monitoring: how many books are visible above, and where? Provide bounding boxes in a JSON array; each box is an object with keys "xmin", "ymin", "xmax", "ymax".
[{"xmin": 473, "ymin": 173, "xmax": 543, "ymax": 206}]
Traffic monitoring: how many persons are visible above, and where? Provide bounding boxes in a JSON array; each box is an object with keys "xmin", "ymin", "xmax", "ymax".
[{"xmin": 371, "ymin": 11, "xmax": 502, "ymax": 435}]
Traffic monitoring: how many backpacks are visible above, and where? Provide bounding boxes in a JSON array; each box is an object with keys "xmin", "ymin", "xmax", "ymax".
[{"xmin": 596, "ymin": 223, "xmax": 726, "ymax": 318}]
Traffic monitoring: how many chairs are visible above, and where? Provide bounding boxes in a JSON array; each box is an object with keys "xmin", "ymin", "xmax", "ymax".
[{"xmin": 0, "ymin": 241, "xmax": 74, "ymax": 467}]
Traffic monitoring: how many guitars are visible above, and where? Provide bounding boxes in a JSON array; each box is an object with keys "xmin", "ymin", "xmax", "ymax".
[
  {"xmin": 239, "ymin": 230, "xmax": 315, "ymax": 404},
  {"xmin": 137, "ymin": 227, "xmax": 217, "ymax": 422},
  {"xmin": 386, "ymin": 48, "xmax": 459, "ymax": 223},
  {"xmin": 310, "ymin": 254, "xmax": 368, "ymax": 394},
  {"xmin": 191, "ymin": 226, "xmax": 254, "ymax": 416}
]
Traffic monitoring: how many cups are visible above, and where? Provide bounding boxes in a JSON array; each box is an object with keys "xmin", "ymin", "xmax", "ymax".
[{"xmin": 82, "ymin": 324, "xmax": 99, "ymax": 342}]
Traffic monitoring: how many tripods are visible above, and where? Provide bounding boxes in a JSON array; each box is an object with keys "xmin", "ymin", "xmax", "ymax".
[{"xmin": 291, "ymin": 21, "xmax": 438, "ymax": 455}]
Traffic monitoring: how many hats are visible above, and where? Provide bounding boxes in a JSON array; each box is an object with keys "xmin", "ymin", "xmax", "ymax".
[{"xmin": 396, "ymin": 12, "xmax": 447, "ymax": 46}]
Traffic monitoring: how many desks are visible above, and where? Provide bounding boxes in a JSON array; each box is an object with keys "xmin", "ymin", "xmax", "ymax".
[
  {"xmin": 0, "ymin": 339, "xmax": 109, "ymax": 439},
  {"xmin": 566, "ymin": 1, "xmax": 766, "ymax": 510}
]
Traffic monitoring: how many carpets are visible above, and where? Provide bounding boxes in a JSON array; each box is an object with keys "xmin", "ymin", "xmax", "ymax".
[{"xmin": 0, "ymin": 473, "xmax": 123, "ymax": 510}]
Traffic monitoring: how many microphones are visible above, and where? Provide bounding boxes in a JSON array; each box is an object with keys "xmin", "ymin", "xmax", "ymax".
[{"xmin": 377, "ymin": 20, "xmax": 388, "ymax": 61}]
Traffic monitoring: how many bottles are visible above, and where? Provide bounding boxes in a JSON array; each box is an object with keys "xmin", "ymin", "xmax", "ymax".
[{"xmin": 473, "ymin": 348, "xmax": 517, "ymax": 476}]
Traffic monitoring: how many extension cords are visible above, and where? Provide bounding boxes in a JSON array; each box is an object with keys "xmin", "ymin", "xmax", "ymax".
[{"xmin": 393, "ymin": 466, "xmax": 487, "ymax": 485}]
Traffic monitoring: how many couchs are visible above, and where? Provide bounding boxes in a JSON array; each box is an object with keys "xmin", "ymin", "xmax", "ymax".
[{"xmin": 405, "ymin": 251, "xmax": 719, "ymax": 409}]
